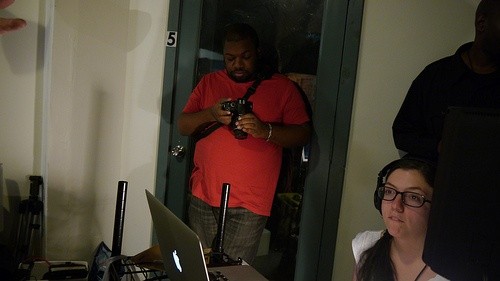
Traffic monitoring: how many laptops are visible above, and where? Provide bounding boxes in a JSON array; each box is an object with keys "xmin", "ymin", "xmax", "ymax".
[{"xmin": 145, "ymin": 189, "xmax": 268, "ymax": 281}]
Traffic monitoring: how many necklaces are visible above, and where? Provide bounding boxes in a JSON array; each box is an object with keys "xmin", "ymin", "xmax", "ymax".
[
  {"xmin": 466, "ymin": 48, "xmax": 480, "ymax": 74},
  {"xmin": 390, "ymin": 262, "xmax": 427, "ymax": 281}
]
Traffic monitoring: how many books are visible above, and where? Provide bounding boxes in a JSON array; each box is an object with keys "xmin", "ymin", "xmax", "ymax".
[{"xmin": 49, "ymin": 265, "xmax": 87, "ymax": 272}]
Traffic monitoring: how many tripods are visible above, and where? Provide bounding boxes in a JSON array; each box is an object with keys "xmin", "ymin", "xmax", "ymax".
[{"xmin": 13, "ymin": 175, "xmax": 43, "ymax": 263}]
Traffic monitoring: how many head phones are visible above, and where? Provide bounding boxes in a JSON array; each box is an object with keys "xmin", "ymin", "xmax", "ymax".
[{"xmin": 373, "ymin": 157, "xmax": 436, "ymax": 210}]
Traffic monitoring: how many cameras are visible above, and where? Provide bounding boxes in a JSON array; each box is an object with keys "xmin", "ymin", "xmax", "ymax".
[{"xmin": 224, "ymin": 99, "xmax": 253, "ymax": 140}]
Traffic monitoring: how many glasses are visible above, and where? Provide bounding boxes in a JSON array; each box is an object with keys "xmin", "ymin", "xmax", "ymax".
[{"xmin": 377, "ymin": 184, "xmax": 434, "ymax": 208}]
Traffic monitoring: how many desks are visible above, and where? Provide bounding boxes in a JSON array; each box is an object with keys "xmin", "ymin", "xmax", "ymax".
[
  {"xmin": 23, "ymin": 256, "xmax": 249, "ymax": 281},
  {"xmin": 271, "ymin": 193, "xmax": 303, "ymax": 254}
]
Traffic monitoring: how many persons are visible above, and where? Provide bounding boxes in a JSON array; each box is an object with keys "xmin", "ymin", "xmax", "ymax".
[
  {"xmin": 389, "ymin": 1, "xmax": 500, "ymax": 159},
  {"xmin": 352, "ymin": 159, "xmax": 451, "ymax": 281},
  {"xmin": 176, "ymin": 24, "xmax": 315, "ymax": 268},
  {"xmin": 0, "ymin": 0, "xmax": 28, "ymax": 33}
]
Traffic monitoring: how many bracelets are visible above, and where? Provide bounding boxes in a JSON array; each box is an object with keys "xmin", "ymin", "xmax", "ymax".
[{"xmin": 265, "ymin": 122, "xmax": 273, "ymax": 143}]
